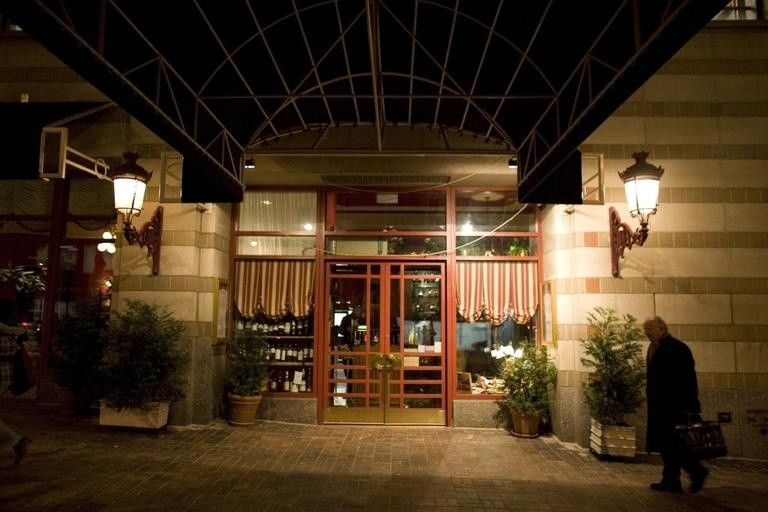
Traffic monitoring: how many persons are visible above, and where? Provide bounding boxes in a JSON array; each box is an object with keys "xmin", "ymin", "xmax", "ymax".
[
  {"xmin": 641, "ymin": 314, "xmax": 711, "ymax": 498},
  {"xmin": 336, "ymin": 303, "xmax": 366, "ymax": 395},
  {"xmin": 0, "ymin": 419, "xmax": 33, "ymax": 468}
]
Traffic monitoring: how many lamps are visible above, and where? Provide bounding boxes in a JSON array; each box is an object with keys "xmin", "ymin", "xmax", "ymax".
[
  {"xmin": 97, "ymin": 227, "xmax": 116, "ymax": 254},
  {"xmin": 243, "ymin": 156, "xmax": 255, "ymax": 168},
  {"xmin": 508, "ymin": 155, "xmax": 519, "ymax": 169},
  {"xmin": 609, "ymin": 149, "xmax": 665, "ymax": 278},
  {"xmin": 113, "ymin": 152, "xmax": 163, "ymax": 275}
]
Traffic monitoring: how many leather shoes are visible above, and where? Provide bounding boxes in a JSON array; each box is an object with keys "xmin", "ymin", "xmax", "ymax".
[
  {"xmin": 689, "ymin": 467, "xmax": 709, "ymax": 490},
  {"xmin": 651, "ymin": 479, "xmax": 682, "ymax": 492}
]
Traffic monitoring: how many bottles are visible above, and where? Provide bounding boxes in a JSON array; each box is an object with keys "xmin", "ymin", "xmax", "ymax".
[{"xmin": 234, "ymin": 318, "xmax": 313, "ymax": 392}]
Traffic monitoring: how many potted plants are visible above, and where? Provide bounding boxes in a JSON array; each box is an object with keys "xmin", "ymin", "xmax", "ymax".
[
  {"xmin": 579, "ymin": 306, "xmax": 647, "ymax": 458},
  {"xmin": 493, "ymin": 342, "xmax": 557, "ymax": 438},
  {"xmin": 221, "ymin": 326, "xmax": 274, "ymax": 426},
  {"xmin": 96, "ymin": 297, "xmax": 191, "ymax": 430}
]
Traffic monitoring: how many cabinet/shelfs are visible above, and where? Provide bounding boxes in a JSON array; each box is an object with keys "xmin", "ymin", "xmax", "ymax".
[{"xmin": 226, "ymin": 304, "xmax": 313, "ymax": 393}]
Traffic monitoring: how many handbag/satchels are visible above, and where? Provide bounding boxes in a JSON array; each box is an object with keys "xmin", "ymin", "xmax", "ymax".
[{"xmin": 675, "ymin": 415, "xmax": 726, "ymax": 460}]
